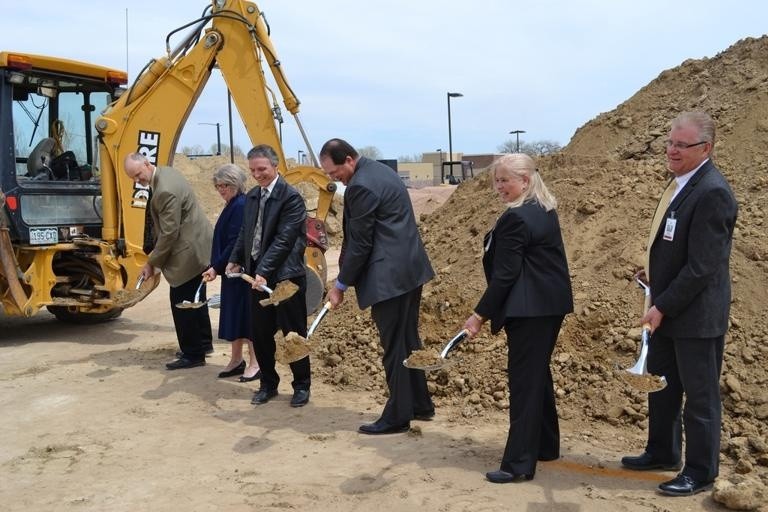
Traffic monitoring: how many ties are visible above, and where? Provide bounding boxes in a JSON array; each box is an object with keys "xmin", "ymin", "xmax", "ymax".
[
  {"xmin": 250, "ymin": 188, "xmax": 267, "ymax": 261},
  {"xmin": 643, "ymin": 178, "xmax": 677, "ymax": 282}
]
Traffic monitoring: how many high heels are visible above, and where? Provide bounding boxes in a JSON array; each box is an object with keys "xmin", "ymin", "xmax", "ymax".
[
  {"xmin": 238, "ymin": 369, "xmax": 261, "ymax": 381},
  {"xmin": 219, "ymin": 360, "xmax": 246, "ymax": 377},
  {"xmin": 486, "ymin": 468, "xmax": 534, "ymax": 482}
]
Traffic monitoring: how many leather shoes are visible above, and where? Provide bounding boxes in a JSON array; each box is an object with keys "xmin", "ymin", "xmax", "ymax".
[
  {"xmin": 414, "ymin": 409, "xmax": 434, "ymax": 419},
  {"xmin": 358, "ymin": 417, "xmax": 409, "ymax": 433},
  {"xmin": 176, "ymin": 351, "xmax": 215, "ymax": 358},
  {"xmin": 166, "ymin": 359, "xmax": 206, "ymax": 370},
  {"xmin": 252, "ymin": 389, "xmax": 277, "ymax": 403},
  {"xmin": 658, "ymin": 472, "xmax": 713, "ymax": 496},
  {"xmin": 291, "ymin": 390, "xmax": 309, "ymax": 406},
  {"xmin": 622, "ymin": 452, "xmax": 682, "ymax": 471}
]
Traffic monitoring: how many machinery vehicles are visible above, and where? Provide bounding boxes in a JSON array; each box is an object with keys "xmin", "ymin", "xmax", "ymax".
[
  {"xmin": 1, "ymin": 1, "xmax": 338, "ymax": 324},
  {"xmin": 435, "ymin": 158, "xmax": 473, "ymax": 187}
]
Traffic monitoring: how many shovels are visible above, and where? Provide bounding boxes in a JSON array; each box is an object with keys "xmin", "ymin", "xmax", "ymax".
[
  {"xmin": 225, "ymin": 266, "xmax": 298, "ymax": 307},
  {"xmin": 274, "ymin": 301, "xmax": 332, "ymax": 365},
  {"xmin": 618, "ymin": 275, "xmax": 668, "ymax": 393},
  {"xmin": 175, "ymin": 274, "xmax": 212, "ymax": 309},
  {"xmin": 403, "ymin": 328, "xmax": 468, "ymax": 371},
  {"xmin": 114, "ymin": 273, "xmax": 149, "ymax": 308}
]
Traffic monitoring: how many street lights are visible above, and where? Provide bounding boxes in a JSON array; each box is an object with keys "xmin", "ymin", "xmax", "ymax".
[
  {"xmin": 445, "ymin": 92, "xmax": 466, "ymax": 185},
  {"xmin": 435, "ymin": 148, "xmax": 443, "ymax": 186},
  {"xmin": 509, "ymin": 127, "xmax": 527, "ymax": 155},
  {"xmin": 197, "ymin": 122, "xmax": 220, "ymax": 157}
]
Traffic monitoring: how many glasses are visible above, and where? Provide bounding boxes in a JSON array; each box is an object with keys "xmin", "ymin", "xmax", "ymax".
[
  {"xmin": 664, "ymin": 140, "xmax": 707, "ymax": 149},
  {"xmin": 215, "ymin": 183, "xmax": 231, "ymax": 187}
]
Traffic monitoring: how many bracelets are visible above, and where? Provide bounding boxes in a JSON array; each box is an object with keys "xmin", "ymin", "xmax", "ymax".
[{"xmin": 472, "ymin": 311, "xmax": 486, "ymax": 324}]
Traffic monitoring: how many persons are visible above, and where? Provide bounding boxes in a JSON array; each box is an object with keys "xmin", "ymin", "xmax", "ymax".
[
  {"xmin": 319, "ymin": 138, "xmax": 436, "ymax": 434},
  {"xmin": 201, "ymin": 163, "xmax": 262, "ymax": 382},
  {"xmin": 462, "ymin": 152, "xmax": 574, "ymax": 483},
  {"xmin": 621, "ymin": 110, "xmax": 739, "ymax": 497},
  {"xmin": 225, "ymin": 144, "xmax": 311, "ymax": 407},
  {"xmin": 125, "ymin": 152, "xmax": 215, "ymax": 370}
]
